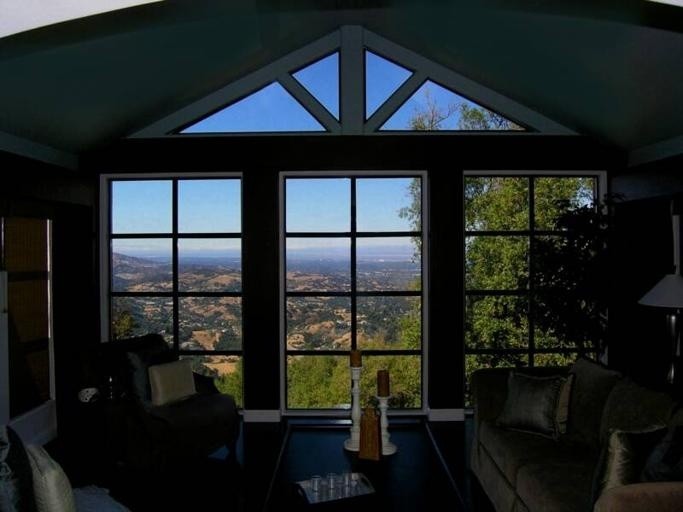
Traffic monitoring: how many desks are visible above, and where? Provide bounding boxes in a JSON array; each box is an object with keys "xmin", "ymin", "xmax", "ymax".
[{"xmin": 263, "ymin": 424, "xmax": 464, "ymax": 512}]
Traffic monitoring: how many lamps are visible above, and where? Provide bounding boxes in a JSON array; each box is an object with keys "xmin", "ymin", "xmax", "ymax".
[{"xmin": 638, "ymin": 215, "xmax": 683, "ymax": 309}]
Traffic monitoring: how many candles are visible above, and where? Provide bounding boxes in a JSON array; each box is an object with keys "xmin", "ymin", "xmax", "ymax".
[
  {"xmin": 377, "ymin": 370, "xmax": 390, "ymax": 397},
  {"xmin": 350, "ymin": 350, "xmax": 362, "ymax": 366}
]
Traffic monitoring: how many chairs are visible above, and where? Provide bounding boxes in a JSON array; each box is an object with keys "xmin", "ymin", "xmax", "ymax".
[
  {"xmin": 101, "ymin": 333, "xmax": 240, "ymax": 461},
  {"xmin": 74, "ymin": 484, "xmax": 131, "ymax": 512}
]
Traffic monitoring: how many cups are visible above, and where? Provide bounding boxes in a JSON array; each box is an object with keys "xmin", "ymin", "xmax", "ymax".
[{"xmin": 311, "ymin": 470, "xmax": 359, "ymax": 495}]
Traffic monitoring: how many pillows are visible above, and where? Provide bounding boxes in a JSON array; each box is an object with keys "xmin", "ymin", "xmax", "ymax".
[
  {"xmin": 26, "ymin": 443, "xmax": 76, "ymax": 512},
  {"xmin": 0, "ymin": 425, "xmax": 36, "ymax": 512},
  {"xmin": 494, "ymin": 358, "xmax": 683, "ymax": 495},
  {"xmin": 148, "ymin": 358, "xmax": 197, "ymax": 408}
]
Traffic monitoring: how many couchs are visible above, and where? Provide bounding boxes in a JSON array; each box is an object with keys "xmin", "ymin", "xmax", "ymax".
[{"xmin": 471, "ymin": 366, "xmax": 683, "ymax": 512}]
